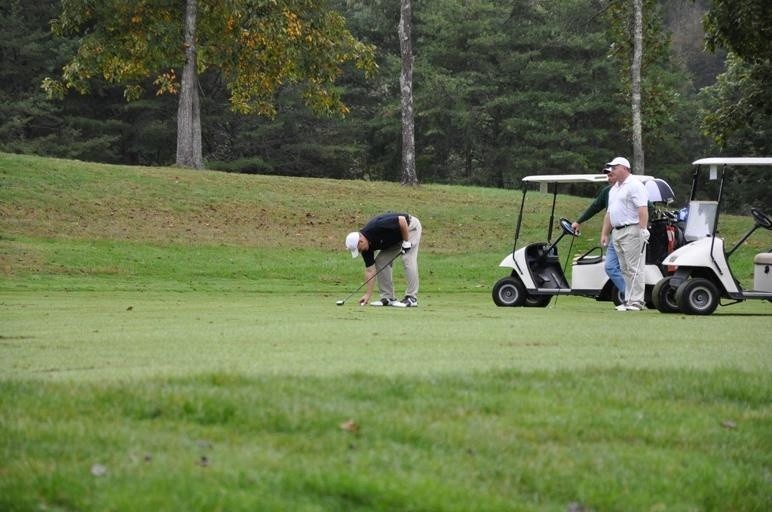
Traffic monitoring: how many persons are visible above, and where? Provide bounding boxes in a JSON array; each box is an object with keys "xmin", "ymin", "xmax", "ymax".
[
  {"xmin": 599, "ymin": 157, "xmax": 650, "ymax": 312},
  {"xmin": 344, "ymin": 211, "xmax": 423, "ymax": 308},
  {"xmin": 571, "ymin": 166, "xmax": 626, "ymax": 299}
]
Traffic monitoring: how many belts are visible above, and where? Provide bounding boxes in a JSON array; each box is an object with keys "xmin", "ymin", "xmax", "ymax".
[{"xmin": 610, "ymin": 222, "xmax": 641, "ymax": 230}]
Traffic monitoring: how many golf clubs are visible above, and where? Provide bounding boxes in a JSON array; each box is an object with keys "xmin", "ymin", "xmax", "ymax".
[
  {"xmin": 665, "ymin": 212, "xmax": 674, "ymax": 226},
  {"xmin": 625, "ymin": 241, "xmax": 645, "ymax": 306},
  {"xmin": 336, "ymin": 251, "xmax": 403, "ymax": 306}
]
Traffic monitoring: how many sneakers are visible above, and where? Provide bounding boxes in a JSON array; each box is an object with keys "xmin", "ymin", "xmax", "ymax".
[
  {"xmin": 614, "ymin": 300, "xmax": 627, "ymax": 311},
  {"xmin": 391, "ymin": 295, "xmax": 418, "ymax": 308},
  {"xmin": 627, "ymin": 301, "xmax": 645, "ymax": 311},
  {"xmin": 369, "ymin": 297, "xmax": 396, "ymax": 306}
]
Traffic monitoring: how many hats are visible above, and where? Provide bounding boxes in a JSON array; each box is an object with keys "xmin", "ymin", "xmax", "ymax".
[
  {"xmin": 603, "ymin": 156, "xmax": 631, "ymax": 174},
  {"xmin": 345, "ymin": 231, "xmax": 360, "ymax": 259}
]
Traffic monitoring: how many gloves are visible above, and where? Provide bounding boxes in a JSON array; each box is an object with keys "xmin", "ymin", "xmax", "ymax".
[
  {"xmin": 400, "ymin": 240, "xmax": 412, "ymax": 254},
  {"xmin": 639, "ymin": 228, "xmax": 651, "ymax": 245}
]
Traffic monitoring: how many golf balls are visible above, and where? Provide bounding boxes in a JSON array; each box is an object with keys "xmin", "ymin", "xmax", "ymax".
[{"xmin": 361, "ymin": 303, "xmax": 364, "ymax": 305}]
